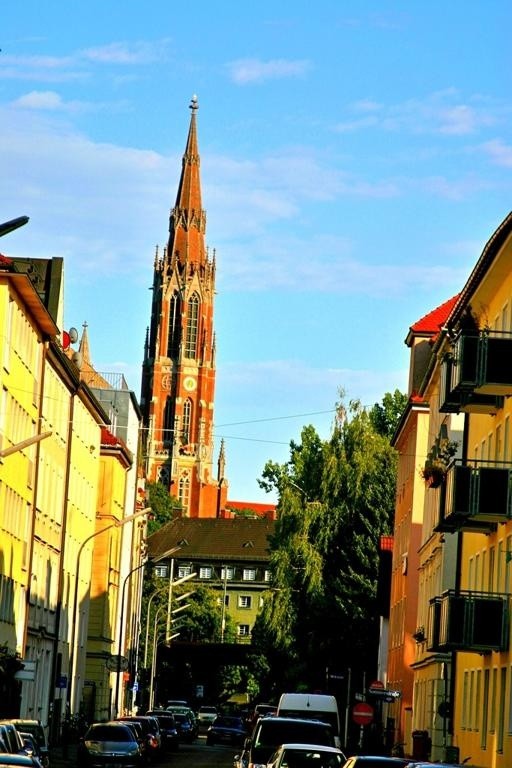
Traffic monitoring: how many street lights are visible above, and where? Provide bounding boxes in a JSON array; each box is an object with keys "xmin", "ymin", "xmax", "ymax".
[
  {"xmin": 62, "ymin": 507, "xmax": 152, "ymax": 759},
  {"xmin": 144, "ymin": 572, "xmax": 197, "ymax": 713},
  {"xmin": 115, "ymin": 547, "xmax": 182, "ymax": 719}
]
